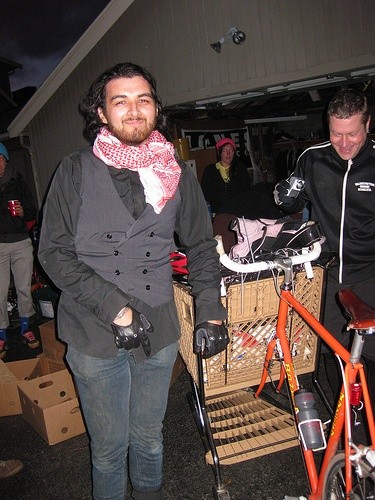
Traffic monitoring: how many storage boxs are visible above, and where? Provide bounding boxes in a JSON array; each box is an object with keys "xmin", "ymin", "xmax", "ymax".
[{"xmin": 0, "ymin": 318, "xmax": 87, "ymax": 446}]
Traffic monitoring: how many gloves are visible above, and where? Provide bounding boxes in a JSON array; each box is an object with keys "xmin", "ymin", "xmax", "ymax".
[
  {"xmin": 110, "ymin": 305, "xmax": 154, "ymax": 357},
  {"xmin": 277, "ymin": 179, "xmax": 296, "ymax": 205},
  {"xmin": 194, "ymin": 318, "xmax": 229, "ymax": 358}
]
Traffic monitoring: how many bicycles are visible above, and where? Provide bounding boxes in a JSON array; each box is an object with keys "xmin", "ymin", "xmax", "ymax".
[{"xmin": 212, "ymin": 232, "xmax": 374, "ymax": 500}]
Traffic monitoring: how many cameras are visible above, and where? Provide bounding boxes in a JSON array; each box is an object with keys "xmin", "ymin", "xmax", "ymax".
[{"xmin": 284, "ymin": 176, "xmax": 305, "ymax": 197}]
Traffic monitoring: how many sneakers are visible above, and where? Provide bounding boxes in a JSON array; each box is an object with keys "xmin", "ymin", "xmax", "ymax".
[
  {"xmin": 0, "ymin": 339, "xmax": 6, "ymax": 359},
  {"xmin": 21, "ymin": 331, "xmax": 39, "ymax": 348}
]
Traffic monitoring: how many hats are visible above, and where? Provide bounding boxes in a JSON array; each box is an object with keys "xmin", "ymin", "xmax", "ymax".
[
  {"xmin": 0, "ymin": 143, "xmax": 9, "ymax": 160},
  {"xmin": 217, "ymin": 138, "xmax": 236, "ymax": 151}
]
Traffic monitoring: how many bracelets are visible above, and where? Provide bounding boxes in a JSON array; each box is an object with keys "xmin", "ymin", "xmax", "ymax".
[{"xmin": 115, "ymin": 307, "xmax": 130, "ymax": 320}]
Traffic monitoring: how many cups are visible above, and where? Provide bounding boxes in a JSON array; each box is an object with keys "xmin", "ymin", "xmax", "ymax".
[
  {"xmin": 8, "ymin": 200, "xmax": 19, "ymax": 216},
  {"xmin": 172, "ymin": 137, "xmax": 190, "ymax": 162}
]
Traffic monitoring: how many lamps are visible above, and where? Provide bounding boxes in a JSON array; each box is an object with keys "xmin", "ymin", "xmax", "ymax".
[{"xmin": 211, "ymin": 26, "xmax": 246, "ymax": 53}]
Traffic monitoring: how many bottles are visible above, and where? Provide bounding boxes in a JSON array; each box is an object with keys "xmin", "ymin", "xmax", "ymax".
[
  {"xmin": 293, "ymin": 384, "xmax": 324, "ymax": 450},
  {"xmin": 38, "ymin": 285, "xmax": 61, "ymax": 319}
]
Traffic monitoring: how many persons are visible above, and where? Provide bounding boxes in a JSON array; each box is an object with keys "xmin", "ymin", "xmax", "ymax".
[
  {"xmin": 0, "ymin": 144, "xmax": 40, "ymax": 357},
  {"xmin": 38, "ymin": 62, "xmax": 230, "ymax": 500},
  {"xmin": 271, "ymin": 88, "xmax": 375, "ymax": 445},
  {"xmin": 201, "ymin": 139, "xmax": 247, "ymax": 217}
]
